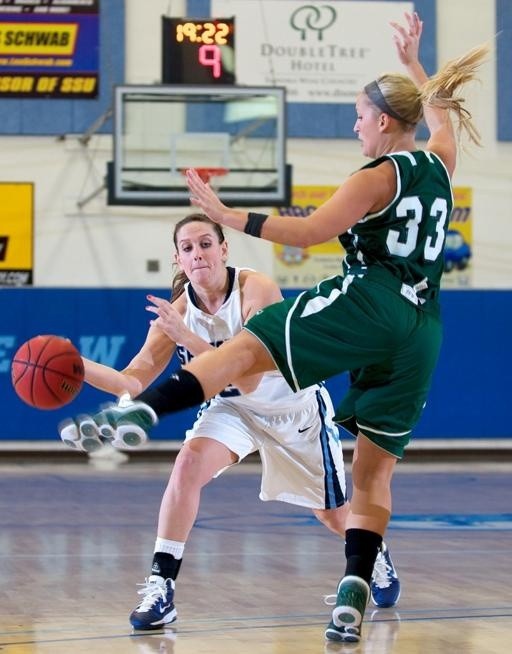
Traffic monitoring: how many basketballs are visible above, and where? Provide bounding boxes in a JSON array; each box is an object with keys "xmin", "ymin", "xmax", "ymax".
[{"xmin": 11, "ymin": 335, "xmax": 84, "ymax": 409}]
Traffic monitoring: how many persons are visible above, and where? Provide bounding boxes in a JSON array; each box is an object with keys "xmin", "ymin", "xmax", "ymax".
[
  {"xmin": 69, "ymin": 212, "xmax": 404, "ymax": 635},
  {"xmin": 59, "ymin": 7, "xmax": 490, "ymax": 643}
]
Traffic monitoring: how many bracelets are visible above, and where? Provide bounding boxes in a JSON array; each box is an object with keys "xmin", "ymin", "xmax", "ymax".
[{"xmin": 243, "ymin": 211, "xmax": 269, "ymax": 240}]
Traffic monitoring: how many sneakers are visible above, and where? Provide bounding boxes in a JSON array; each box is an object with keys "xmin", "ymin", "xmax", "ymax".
[
  {"xmin": 366, "ymin": 543, "xmax": 401, "ymax": 609},
  {"xmin": 127, "ymin": 572, "xmax": 179, "ymax": 631},
  {"xmin": 324, "ymin": 573, "xmax": 370, "ymax": 644},
  {"xmin": 57, "ymin": 391, "xmax": 160, "ymax": 456}
]
